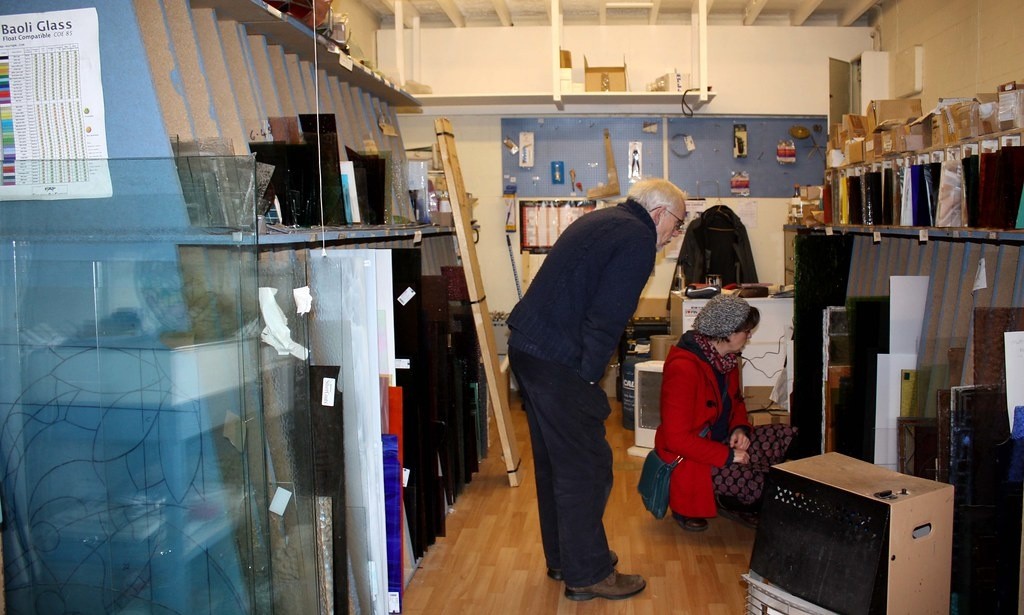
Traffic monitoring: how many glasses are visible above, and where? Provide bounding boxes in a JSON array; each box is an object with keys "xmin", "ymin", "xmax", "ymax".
[{"xmin": 649, "ymin": 205, "xmax": 685, "ymax": 231}]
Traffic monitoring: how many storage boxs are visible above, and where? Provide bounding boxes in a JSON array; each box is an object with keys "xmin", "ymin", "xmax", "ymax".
[
  {"xmin": 744, "ymin": 386, "xmax": 791, "ymax": 426},
  {"xmin": 488, "ymin": 312, "xmax": 509, "ymax": 354},
  {"xmin": 826, "ymin": 82, "xmax": 1024, "ymax": 171}
]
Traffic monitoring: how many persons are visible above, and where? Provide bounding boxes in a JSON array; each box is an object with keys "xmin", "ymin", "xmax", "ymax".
[
  {"xmin": 655, "ymin": 294, "xmax": 799, "ymax": 532},
  {"xmin": 505, "ymin": 178, "xmax": 688, "ymax": 600}
]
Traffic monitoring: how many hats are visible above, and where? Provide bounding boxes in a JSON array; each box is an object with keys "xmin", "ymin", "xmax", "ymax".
[{"xmin": 691, "ymin": 293, "xmax": 751, "ymax": 338}]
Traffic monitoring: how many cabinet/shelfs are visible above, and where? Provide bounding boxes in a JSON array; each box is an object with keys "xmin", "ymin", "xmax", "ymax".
[{"xmin": 0, "ymin": 0, "xmax": 459, "ymax": 302}]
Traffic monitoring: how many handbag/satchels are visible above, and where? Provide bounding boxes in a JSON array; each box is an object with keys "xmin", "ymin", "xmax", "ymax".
[{"xmin": 636, "ymin": 372, "xmax": 730, "ymax": 521}]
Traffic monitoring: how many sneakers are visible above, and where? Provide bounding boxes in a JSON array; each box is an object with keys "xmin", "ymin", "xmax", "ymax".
[
  {"xmin": 671, "ymin": 510, "xmax": 707, "ymax": 532},
  {"xmin": 546, "ymin": 549, "xmax": 618, "ymax": 581},
  {"xmin": 564, "ymin": 569, "xmax": 646, "ymax": 602},
  {"xmin": 714, "ymin": 494, "xmax": 758, "ymax": 528}
]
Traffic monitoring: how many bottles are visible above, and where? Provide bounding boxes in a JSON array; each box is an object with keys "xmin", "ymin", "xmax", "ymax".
[{"xmin": 623, "ymin": 339, "xmax": 651, "ymax": 430}]
